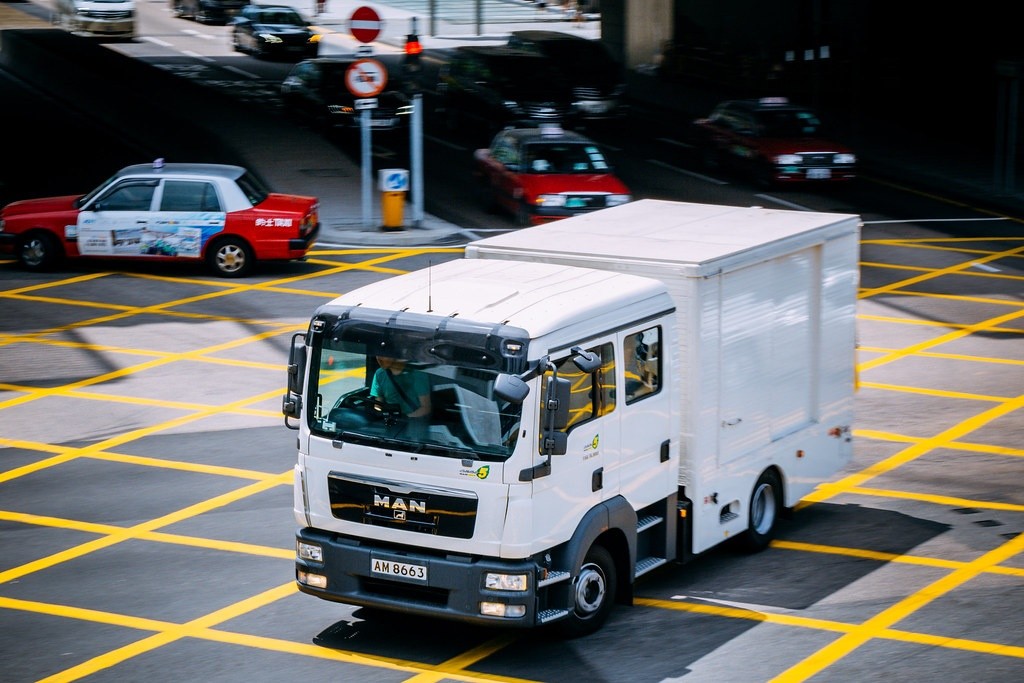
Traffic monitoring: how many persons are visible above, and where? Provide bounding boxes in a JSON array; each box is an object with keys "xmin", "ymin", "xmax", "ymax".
[
  {"xmin": 458, "ymin": 392, "xmax": 521, "ymax": 446},
  {"xmin": 368, "ymin": 354, "xmax": 432, "ymax": 420},
  {"xmin": 118, "ymin": 185, "xmax": 150, "ymax": 211}
]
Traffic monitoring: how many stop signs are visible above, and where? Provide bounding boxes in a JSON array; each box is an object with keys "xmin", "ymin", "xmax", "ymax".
[{"xmin": 348, "ymin": 4, "xmax": 382, "ymax": 44}]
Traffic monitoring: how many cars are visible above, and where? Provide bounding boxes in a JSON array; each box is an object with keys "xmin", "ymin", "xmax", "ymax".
[
  {"xmin": 60, "ymin": 0, "xmax": 134, "ymax": 40},
  {"xmin": 283, "ymin": 54, "xmax": 412, "ymax": 152},
  {"xmin": 233, "ymin": 3, "xmax": 320, "ymax": 60},
  {"xmin": 399, "ymin": 30, "xmax": 628, "ymax": 152},
  {"xmin": 1, "ymin": 157, "xmax": 323, "ymax": 279},
  {"xmin": 474, "ymin": 121, "xmax": 635, "ymax": 228},
  {"xmin": 169, "ymin": 0, "xmax": 253, "ymax": 26},
  {"xmin": 688, "ymin": 95, "xmax": 865, "ymax": 196}
]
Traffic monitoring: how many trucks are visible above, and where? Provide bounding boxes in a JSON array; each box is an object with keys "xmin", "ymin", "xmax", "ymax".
[{"xmin": 281, "ymin": 200, "xmax": 862, "ymax": 639}]
